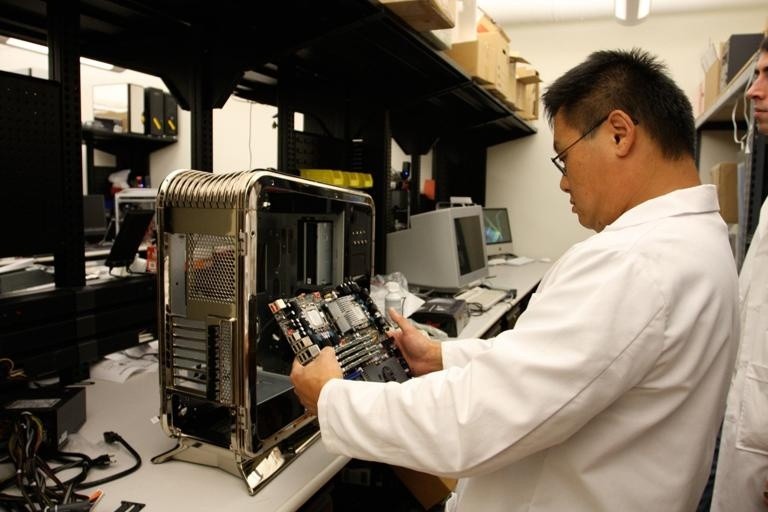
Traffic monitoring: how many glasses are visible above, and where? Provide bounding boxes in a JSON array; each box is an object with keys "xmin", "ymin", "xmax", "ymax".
[{"xmin": 549, "ymin": 112, "xmax": 639, "ymax": 178}]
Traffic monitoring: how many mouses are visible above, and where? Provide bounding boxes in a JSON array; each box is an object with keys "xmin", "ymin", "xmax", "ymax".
[{"xmin": 539, "ymin": 257, "xmax": 551, "ymax": 263}]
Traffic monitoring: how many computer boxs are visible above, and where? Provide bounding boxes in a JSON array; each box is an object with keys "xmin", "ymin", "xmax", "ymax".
[
  {"xmin": 151, "ymin": 168, "xmax": 376, "ymax": 496},
  {"xmin": 144, "ymin": 87, "xmax": 164, "ymax": 135},
  {"xmin": 163, "ymin": 92, "xmax": 178, "ymax": 136}
]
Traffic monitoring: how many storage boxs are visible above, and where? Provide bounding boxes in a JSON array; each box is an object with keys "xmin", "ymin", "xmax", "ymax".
[
  {"xmin": 693, "ymin": 34, "xmax": 768, "ymax": 120},
  {"xmin": 708, "ymin": 162, "xmax": 745, "ymax": 229},
  {"xmin": 377, "ymin": 0, "xmax": 543, "ymax": 121}
]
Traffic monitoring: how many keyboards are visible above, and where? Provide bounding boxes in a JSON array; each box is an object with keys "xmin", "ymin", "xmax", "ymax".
[
  {"xmin": 455, "ymin": 288, "xmax": 505, "ymax": 309},
  {"xmin": 504, "ymin": 255, "xmax": 533, "ymax": 266}
]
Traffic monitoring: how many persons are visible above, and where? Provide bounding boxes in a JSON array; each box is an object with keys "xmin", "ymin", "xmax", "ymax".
[
  {"xmin": 709, "ymin": 34, "xmax": 768, "ymax": 511},
  {"xmin": 286, "ymin": 45, "xmax": 744, "ymax": 511}
]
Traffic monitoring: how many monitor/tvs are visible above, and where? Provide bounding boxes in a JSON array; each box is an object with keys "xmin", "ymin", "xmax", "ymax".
[
  {"xmin": 481, "ymin": 207, "xmax": 514, "ymax": 257},
  {"xmin": 83, "ymin": 195, "xmax": 107, "ymax": 236},
  {"xmin": 385, "ymin": 205, "xmax": 489, "ymax": 294}
]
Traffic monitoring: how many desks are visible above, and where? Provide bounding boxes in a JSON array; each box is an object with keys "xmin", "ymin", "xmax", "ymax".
[{"xmin": 0, "ymin": 254, "xmax": 554, "ymax": 512}]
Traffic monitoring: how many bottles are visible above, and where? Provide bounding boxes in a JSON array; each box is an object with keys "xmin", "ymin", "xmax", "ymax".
[{"xmin": 384, "ymin": 282, "xmax": 404, "ymax": 330}]
